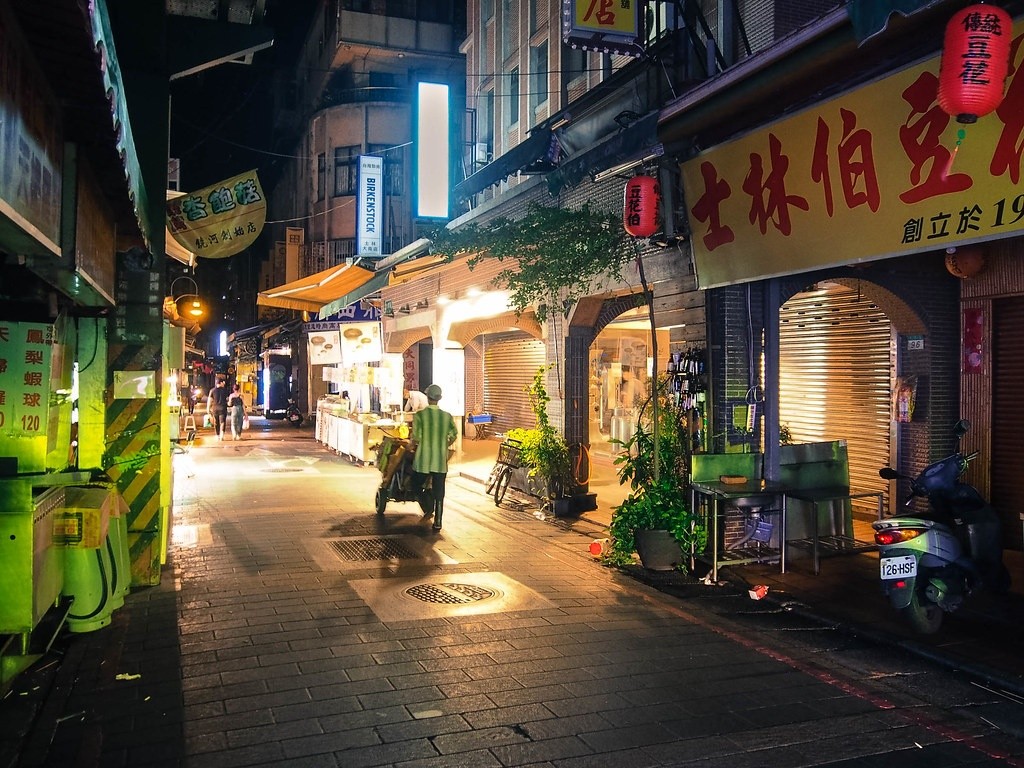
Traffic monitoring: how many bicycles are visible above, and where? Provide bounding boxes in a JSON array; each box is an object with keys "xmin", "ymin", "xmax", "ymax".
[{"xmin": 484, "ymin": 437, "xmax": 524, "ymax": 505}]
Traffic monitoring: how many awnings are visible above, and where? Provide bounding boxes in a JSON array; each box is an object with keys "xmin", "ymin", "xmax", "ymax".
[
  {"xmin": 258, "ymin": 250, "xmax": 473, "ymax": 321},
  {"xmin": 448, "ymin": 127, "xmax": 550, "ymax": 204}
]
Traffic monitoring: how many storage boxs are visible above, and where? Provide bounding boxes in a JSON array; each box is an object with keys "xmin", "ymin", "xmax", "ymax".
[
  {"xmin": 467, "ymin": 414, "xmax": 492, "ymax": 423},
  {"xmin": 63, "ymin": 488, "xmax": 110, "ymax": 549}
]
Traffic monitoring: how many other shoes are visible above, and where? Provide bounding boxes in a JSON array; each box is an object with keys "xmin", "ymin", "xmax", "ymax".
[
  {"xmin": 423, "ymin": 508, "xmax": 434, "ymax": 517},
  {"xmin": 432, "ymin": 524, "xmax": 441, "ymax": 530}
]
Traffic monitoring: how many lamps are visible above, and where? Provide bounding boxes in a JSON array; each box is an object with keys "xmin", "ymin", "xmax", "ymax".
[
  {"xmin": 170, "ymin": 277, "xmax": 200, "ymax": 308},
  {"xmin": 175, "ymin": 295, "xmax": 202, "ymax": 315}
]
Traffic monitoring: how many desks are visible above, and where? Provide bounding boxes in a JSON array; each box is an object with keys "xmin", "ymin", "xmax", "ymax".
[
  {"xmin": 688, "ymin": 482, "xmax": 790, "ymax": 582},
  {"xmin": 780, "ymin": 488, "xmax": 884, "ymax": 575},
  {"xmin": 314, "ymin": 408, "xmax": 401, "ymax": 468},
  {"xmin": 467, "ymin": 421, "xmax": 493, "ymax": 442}
]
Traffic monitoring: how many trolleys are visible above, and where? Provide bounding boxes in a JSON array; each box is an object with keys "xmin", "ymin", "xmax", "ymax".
[{"xmin": 373, "ymin": 447, "xmax": 435, "ymax": 517}]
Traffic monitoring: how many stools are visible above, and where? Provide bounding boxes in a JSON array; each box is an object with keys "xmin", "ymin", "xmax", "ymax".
[{"xmin": 184, "ymin": 416, "xmax": 197, "ymax": 431}]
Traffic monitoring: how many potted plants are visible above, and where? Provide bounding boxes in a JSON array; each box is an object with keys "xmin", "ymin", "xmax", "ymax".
[
  {"xmin": 498, "ymin": 362, "xmax": 579, "ymax": 498},
  {"xmin": 419, "ymin": 200, "xmax": 707, "ymax": 572}
]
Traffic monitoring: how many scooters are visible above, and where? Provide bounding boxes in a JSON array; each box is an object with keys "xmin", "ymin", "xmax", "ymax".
[
  {"xmin": 279, "ymin": 394, "xmax": 303, "ymax": 428},
  {"xmin": 876, "ymin": 417, "xmax": 1012, "ymax": 636}
]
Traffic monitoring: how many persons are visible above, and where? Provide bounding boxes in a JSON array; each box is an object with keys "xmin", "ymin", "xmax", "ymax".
[
  {"xmin": 410, "ymin": 385, "xmax": 457, "ymax": 533},
  {"xmin": 228, "ymin": 384, "xmax": 248, "ymax": 440},
  {"xmin": 207, "ymin": 378, "xmax": 229, "ymax": 441},
  {"xmin": 403, "ymin": 389, "xmax": 428, "ymax": 412},
  {"xmin": 186, "ymin": 385, "xmax": 195, "ymax": 414},
  {"xmin": 621, "ymin": 367, "xmax": 645, "ymax": 406}
]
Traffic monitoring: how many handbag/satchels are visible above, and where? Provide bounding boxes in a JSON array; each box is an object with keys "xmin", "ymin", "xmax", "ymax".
[
  {"xmin": 242, "ymin": 419, "xmax": 249, "ymax": 429},
  {"xmin": 203, "ymin": 414, "xmax": 212, "ymax": 427}
]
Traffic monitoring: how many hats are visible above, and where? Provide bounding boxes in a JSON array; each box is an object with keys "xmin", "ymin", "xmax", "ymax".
[{"xmin": 424, "ymin": 385, "xmax": 442, "ymax": 400}]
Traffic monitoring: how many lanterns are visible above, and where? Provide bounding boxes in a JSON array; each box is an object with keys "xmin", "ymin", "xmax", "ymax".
[
  {"xmin": 937, "ymin": 4, "xmax": 1012, "ymax": 180},
  {"xmin": 624, "ymin": 176, "xmax": 662, "ymax": 239}
]
